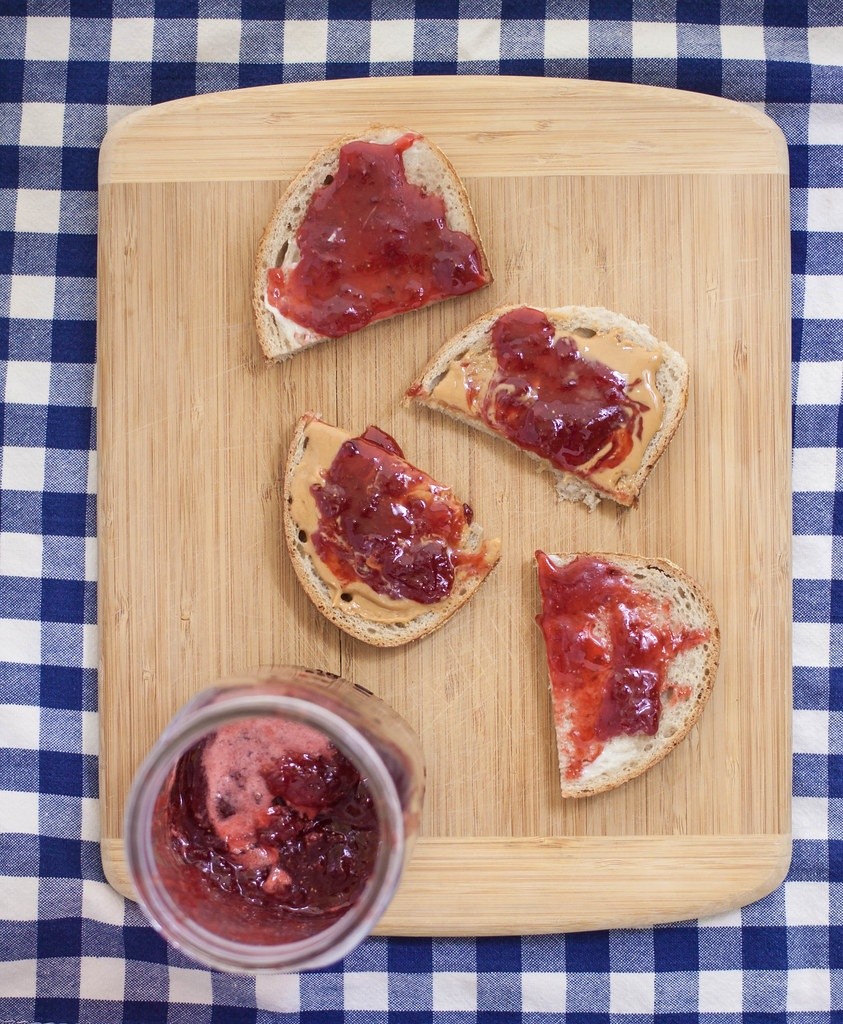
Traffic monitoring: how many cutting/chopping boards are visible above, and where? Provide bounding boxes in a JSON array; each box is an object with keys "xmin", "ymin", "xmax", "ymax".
[{"xmin": 97, "ymin": 74, "xmax": 793, "ymax": 937}]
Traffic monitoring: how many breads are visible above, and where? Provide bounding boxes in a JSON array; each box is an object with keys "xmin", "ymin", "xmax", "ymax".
[
  {"xmin": 532, "ymin": 550, "xmax": 721, "ymax": 798},
  {"xmin": 282, "ymin": 410, "xmax": 502, "ymax": 649},
  {"xmin": 399, "ymin": 303, "xmax": 690, "ymax": 513},
  {"xmin": 252, "ymin": 122, "xmax": 494, "ymax": 368}
]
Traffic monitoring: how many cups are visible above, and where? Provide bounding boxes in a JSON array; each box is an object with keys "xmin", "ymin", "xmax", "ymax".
[{"xmin": 120, "ymin": 665, "xmax": 427, "ymax": 978}]
